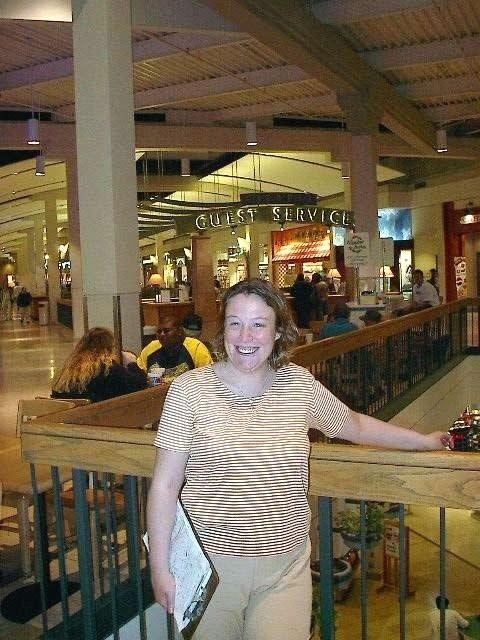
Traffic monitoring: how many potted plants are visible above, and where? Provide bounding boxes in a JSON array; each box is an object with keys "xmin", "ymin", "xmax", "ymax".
[{"xmin": 335, "ymin": 502, "xmax": 385, "ymax": 551}]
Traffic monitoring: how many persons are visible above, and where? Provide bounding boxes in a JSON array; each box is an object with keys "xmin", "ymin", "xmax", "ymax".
[
  {"xmin": 290, "ymin": 272, "xmax": 328, "ymax": 328},
  {"xmin": 321, "ymin": 302, "xmax": 359, "ymax": 338},
  {"xmin": 428, "ymin": 596, "xmax": 469, "ymax": 640},
  {"xmin": 411, "ymin": 268, "xmax": 441, "ymax": 309},
  {"xmin": 17, "ymin": 287, "xmax": 33, "ymax": 323},
  {"xmin": 358, "ymin": 310, "xmax": 382, "ymax": 330},
  {"xmin": 51, "ymin": 327, "xmax": 149, "ymax": 403},
  {"xmin": 145, "ymin": 277, "xmax": 456, "ymax": 640},
  {"xmin": 135, "ymin": 314, "xmax": 214, "ymax": 380},
  {"xmin": 390, "ymin": 266, "xmax": 400, "ymax": 292}
]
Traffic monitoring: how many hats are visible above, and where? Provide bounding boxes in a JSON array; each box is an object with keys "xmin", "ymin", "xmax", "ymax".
[
  {"xmin": 359, "ymin": 310, "xmax": 382, "ymax": 321},
  {"xmin": 183, "ymin": 314, "xmax": 202, "ymax": 330}
]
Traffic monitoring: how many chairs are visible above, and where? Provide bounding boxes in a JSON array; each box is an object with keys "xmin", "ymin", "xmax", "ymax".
[
  {"xmin": 0, "ymin": 396, "xmax": 124, "ymax": 574},
  {"xmin": 297, "ymin": 296, "xmax": 443, "ymax": 414}
]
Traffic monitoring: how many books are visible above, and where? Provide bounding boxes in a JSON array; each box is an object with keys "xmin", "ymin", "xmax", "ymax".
[{"xmin": 142, "ymin": 496, "xmax": 219, "ymax": 640}]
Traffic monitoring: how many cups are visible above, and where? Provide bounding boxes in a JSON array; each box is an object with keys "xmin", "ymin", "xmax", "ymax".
[{"xmin": 149, "ymin": 368, "xmax": 166, "ymax": 385}]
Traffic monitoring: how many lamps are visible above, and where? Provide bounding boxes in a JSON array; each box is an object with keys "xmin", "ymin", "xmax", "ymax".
[
  {"xmin": 25, "ymin": 37, "xmax": 45, "ymax": 176},
  {"xmin": 326, "ymin": 268, "xmax": 341, "ymax": 295},
  {"xmin": 342, "ymin": 161, "xmax": 350, "ymax": 179},
  {"xmin": 181, "ymin": 121, "xmax": 191, "ymax": 177},
  {"xmin": 245, "ymin": 118, "xmax": 257, "ymax": 143},
  {"xmin": 380, "ymin": 266, "xmax": 394, "ymax": 291},
  {"xmin": 458, "ymin": 202, "xmax": 475, "ymax": 224},
  {"xmin": 145, "ymin": 274, "xmax": 166, "ymax": 303},
  {"xmin": 436, "ymin": 65, "xmax": 448, "ymax": 152}
]
